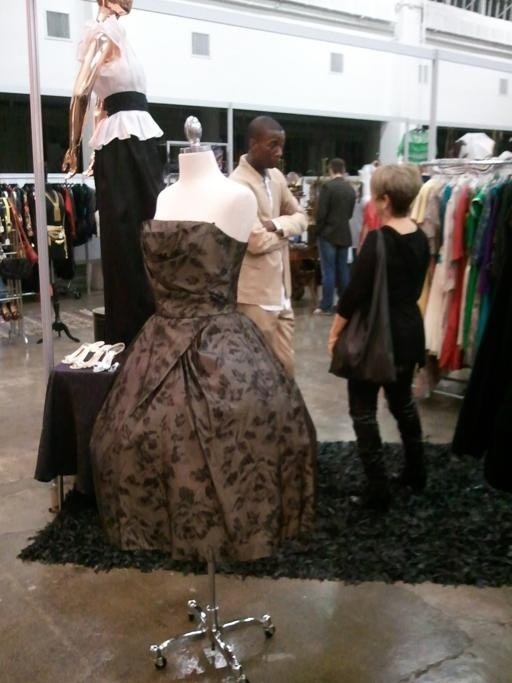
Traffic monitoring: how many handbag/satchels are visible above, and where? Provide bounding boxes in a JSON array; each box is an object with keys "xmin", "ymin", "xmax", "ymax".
[
  {"xmin": 328, "ymin": 239, "xmax": 398, "ymax": 388},
  {"xmin": 1, "ymin": 242, "xmax": 36, "ymax": 283}
]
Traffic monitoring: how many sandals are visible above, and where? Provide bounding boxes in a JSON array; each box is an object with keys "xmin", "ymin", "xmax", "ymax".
[{"xmin": 60, "ymin": 340, "xmax": 126, "ymax": 374}]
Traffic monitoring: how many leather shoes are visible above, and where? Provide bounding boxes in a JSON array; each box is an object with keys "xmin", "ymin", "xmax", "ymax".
[
  {"xmin": 350, "ymin": 494, "xmax": 388, "ymax": 511},
  {"xmin": 393, "ymin": 472, "xmax": 426, "ymax": 489},
  {"xmin": 2, "ymin": 303, "xmax": 20, "ymax": 321}
]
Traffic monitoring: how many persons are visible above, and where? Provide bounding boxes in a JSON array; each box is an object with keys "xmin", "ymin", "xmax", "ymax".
[
  {"xmin": 229, "ymin": 112, "xmax": 309, "ymax": 385},
  {"xmin": 62, "ymin": 0, "xmax": 169, "ymax": 345},
  {"xmin": 326, "ymin": 159, "xmax": 433, "ymax": 500},
  {"xmin": 313, "ymin": 156, "xmax": 357, "ymax": 315},
  {"xmin": 90, "ymin": 111, "xmax": 319, "ymax": 568}
]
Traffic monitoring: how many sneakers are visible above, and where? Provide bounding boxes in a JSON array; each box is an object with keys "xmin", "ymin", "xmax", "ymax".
[{"xmin": 314, "ymin": 308, "xmax": 334, "ymax": 315}]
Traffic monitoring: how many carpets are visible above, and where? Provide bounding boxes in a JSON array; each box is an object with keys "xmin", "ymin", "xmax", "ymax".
[{"xmin": 18, "ymin": 442, "xmax": 512, "ymax": 589}]
